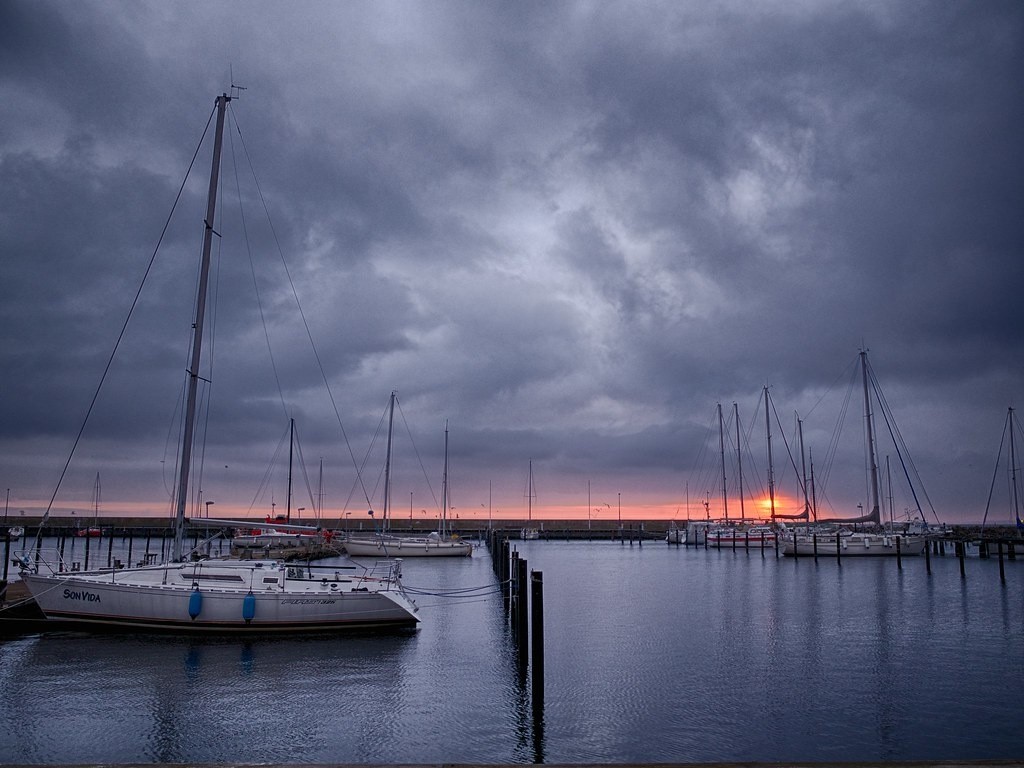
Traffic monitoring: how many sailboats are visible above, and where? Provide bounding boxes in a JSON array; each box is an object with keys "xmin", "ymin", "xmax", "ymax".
[
  {"xmin": 10, "ymin": 62, "xmax": 422, "ymax": 633},
  {"xmin": 665, "ymin": 337, "xmax": 953, "ymax": 555},
  {"xmin": 329, "ymin": 391, "xmax": 472, "ymax": 556},
  {"xmin": 78, "ymin": 471, "xmax": 106, "ymax": 537},
  {"xmin": 979, "ymin": 407, "xmax": 1024, "ymax": 554},
  {"xmin": 232, "ymin": 420, "xmax": 346, "ymax": 547}
]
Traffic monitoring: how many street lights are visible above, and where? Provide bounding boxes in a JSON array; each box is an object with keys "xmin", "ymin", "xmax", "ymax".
[
  {"xmin": 411, "ymin": 492, "xmax": 413, "ymax": 527},
  {"xmin": 206, "ymin": 501, "xmax": 214, "ymax": 555},
  {"xmin": 618, "ymin": 493, "xmax": 620, "ymax": 530},
  {"xmin": 346, "ymin": 513, "xmax": 352, "ymax": 537},
  {"xmin": 298, "ymin": 508, "xmax": 305, "ymax": 525}
]
